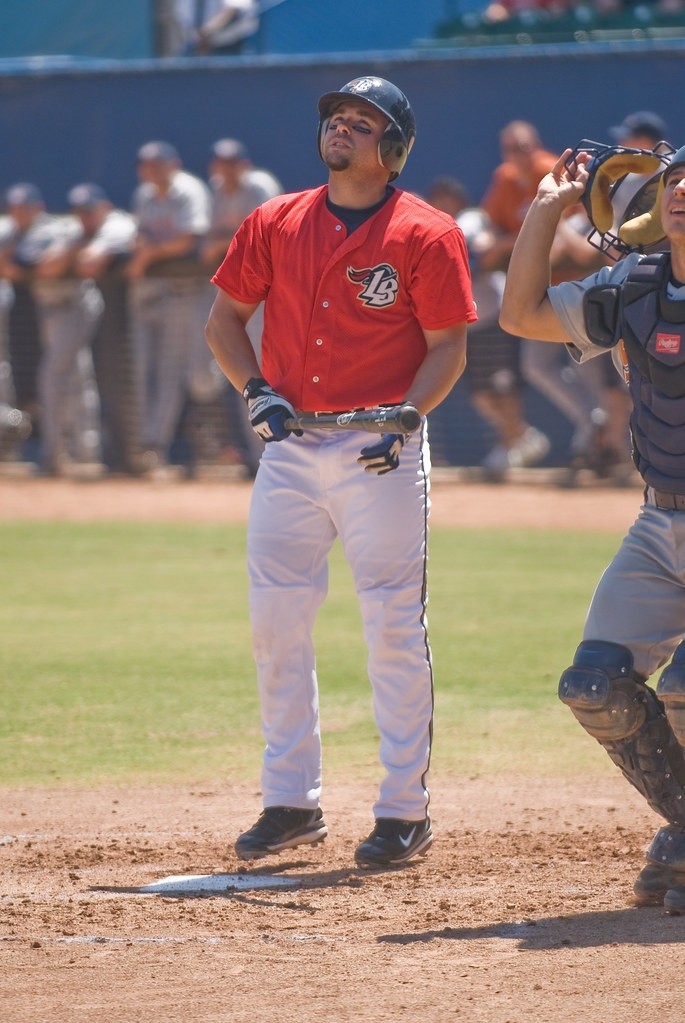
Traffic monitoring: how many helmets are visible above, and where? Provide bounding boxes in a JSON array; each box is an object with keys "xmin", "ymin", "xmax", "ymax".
[
  {"xmin": 662, "ymin": 144, "xmax": 685, "ymax": 187},
  {"xmin": 316, "ymin": 76, "xmax": 417, "ymax": 182}
]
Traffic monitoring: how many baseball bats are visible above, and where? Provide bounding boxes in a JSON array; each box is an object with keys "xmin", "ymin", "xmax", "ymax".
[{"xmin": 284, "ymin": 404, "xmax": 421, "ymax": 435}]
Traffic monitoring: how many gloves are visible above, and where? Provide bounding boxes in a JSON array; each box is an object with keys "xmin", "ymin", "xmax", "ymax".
[
  {"xmin": 357, "ymin": 430, "xmax": 413, "ymax": 477},
  {"xmin": 241, "ymin": 378, "xmax": 304, "ymax": 442}
]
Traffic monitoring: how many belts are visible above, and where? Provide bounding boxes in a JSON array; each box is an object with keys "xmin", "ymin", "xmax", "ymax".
[
  {"xmin": 644, "ymin": 487, "xmax": 684, "ymax": 512},
  {"xmin": 307, "ymin": 402, "xmax": 403, "ymax": 419}
]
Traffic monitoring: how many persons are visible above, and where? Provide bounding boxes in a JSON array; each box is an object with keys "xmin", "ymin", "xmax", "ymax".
[
  {"xmin": 0, "ymin": 138, "xmax": 284, "ymax": 476},
  {"xmin": 162, "ymin": 0, "xmax": 259, "ymax": 57},
  {"xmin": 500, "ymin": 139, "xmax": 685, "ymax": 918},
  {"xmin": 204, "ymin": 75, "xmax": 481, "ymax": 870},
  {"xmin": 430, "ymin": 112, "xmax": 668, "ymax": 467}
]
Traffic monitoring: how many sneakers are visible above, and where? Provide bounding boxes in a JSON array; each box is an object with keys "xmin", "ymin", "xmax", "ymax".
[
  {"xmin": 236, "ymin": 805, "xmax": 328, "ymax": 860},
  {"xmin": 355, "ymin": 815, "xmax": 433, "ymax": 869},
  {"xmin": 634, "ymin": 827, "xmax": 685, "ymax": 913}
]
